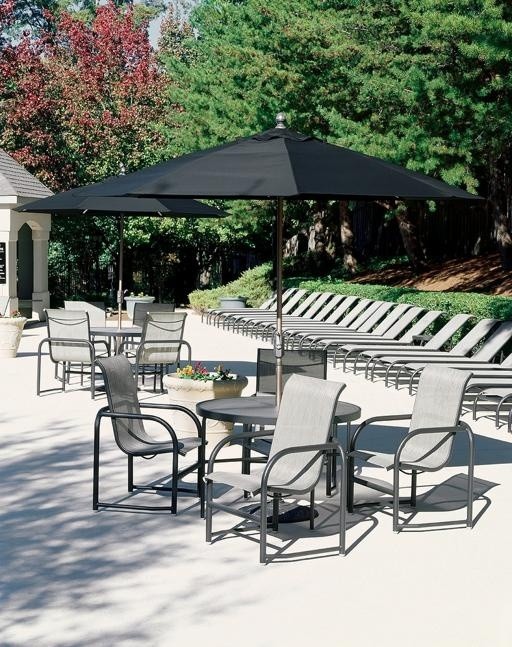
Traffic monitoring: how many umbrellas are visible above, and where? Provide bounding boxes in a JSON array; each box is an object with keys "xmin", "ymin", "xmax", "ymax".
[
  {"xmin": 73, "ymin": 113, "xmax": 487, "ymax": 411},
  {"xmin": 11, "ymin": 168, "xmax": 231, "ymax": 354}
]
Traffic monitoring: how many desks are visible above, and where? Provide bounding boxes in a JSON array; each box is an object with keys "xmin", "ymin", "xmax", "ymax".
[
  {"xmin": 87, "ymin": 324, "xmax": 145, "ymax": 393},
  {"xmin": 192, "ymin": 393, "xmax": 363, "ymax": 533}
]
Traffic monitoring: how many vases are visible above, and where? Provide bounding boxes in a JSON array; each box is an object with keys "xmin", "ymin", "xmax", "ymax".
[{"xmin": 0, "ymin": 317, "xmax": 27, "ymax": 358}]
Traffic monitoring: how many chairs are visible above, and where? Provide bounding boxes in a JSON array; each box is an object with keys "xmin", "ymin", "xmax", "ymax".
[
  {"xmin": 118, "ymin": 311, "xmax": 188, "ymax": 394},
  {"xmin": 199, "ymin": 287, "xmax": 512, "ymax": 432},
  {"xmin": 63, "ymin": 299, "xmax": 108, "ymax": 327},
  {"xmin": 37, "ymin": 305, "xmax": 108, "ymax": 399},
  {"xmin": 203, "ymin": 371, "xmax": 352, "ymax": 562},
  {"xmin": 132, "ymin": 301, "xmax": 175, "ymax": 325},
  {"xmin": 92, "ymin": 350, "xmax": 204, "ymax": 519},
  {"xmin": 246, "ymin": 346, "xmax": 337, "ymax": 492},
  {"xmin": 346, "ymin": 365, "xmax": 480, "ymax": 535}
]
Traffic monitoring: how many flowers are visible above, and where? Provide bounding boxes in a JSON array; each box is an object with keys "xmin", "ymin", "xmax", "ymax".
[
  {"xmin": 176, "ymin": 360, "xmax": 231, "ymax": 381},
  {"xmin": 0, "ymin": 310, "xmax": 20, "ymax": 318}
]
